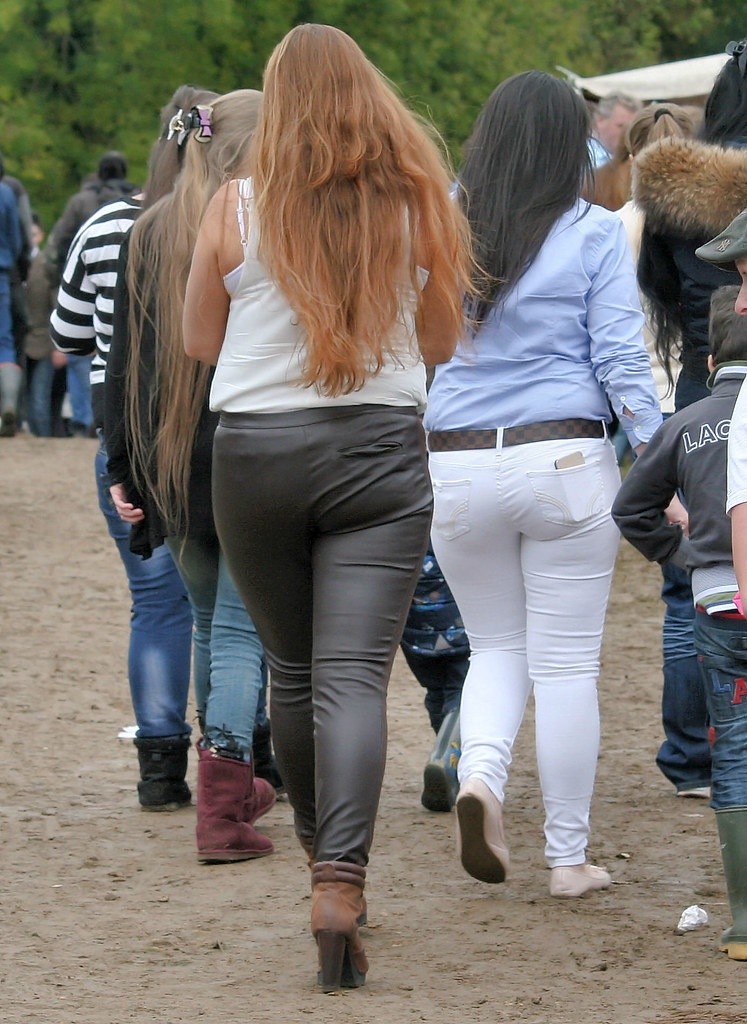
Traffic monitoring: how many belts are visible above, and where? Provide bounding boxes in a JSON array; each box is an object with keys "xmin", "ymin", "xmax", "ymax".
[{"xmin": 426, "ymin": 419, "xmax": 611, "ymax": 452}]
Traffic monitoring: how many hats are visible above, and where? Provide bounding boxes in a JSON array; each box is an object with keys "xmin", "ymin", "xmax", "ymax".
[{"xmin": 694, "ymin": 205, "xmax": 747, "ymax": 273}]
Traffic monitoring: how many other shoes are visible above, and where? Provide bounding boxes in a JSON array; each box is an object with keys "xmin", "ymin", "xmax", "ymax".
[
  {"xmin": 549, "ymin": 864, "xmax": 610, "ymax": 898},
  {"xmin": 453, "ymin": 778, "xmax": 510, "ymax": 885}
]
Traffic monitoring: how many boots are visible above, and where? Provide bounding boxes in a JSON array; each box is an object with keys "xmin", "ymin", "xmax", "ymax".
[
  {"xmin": 132, "ymin": 729, "xmax": 193, "ymax": 813},
  {"xmin": 715, "ymin": 805, "xmax": 747, "ymax": 961},
  {"xmin": 308, "ymin": 859, "xmax": 370, "ymax": 995},
  {"xmin": 420, "ymin": 707, "xmax": 464, "ymax": 814},
  {"xmin": 1, "ymin": 362, "xmax": 21, "ymax": 437},
  {"xmin": 195, "ymin": 720, "xmax": 285, "ymax": 861}
]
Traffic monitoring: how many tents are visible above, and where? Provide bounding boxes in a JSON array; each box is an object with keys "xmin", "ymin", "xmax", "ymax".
[{"xmin": 561, "ymin": 53, "xmax": 737, "ymax": 107}]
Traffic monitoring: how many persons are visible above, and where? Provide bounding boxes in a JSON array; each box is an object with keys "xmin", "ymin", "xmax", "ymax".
[
  {"xmin": 181, "ymin": 23, "xmax": 490, "ymax": 992},
  {"xmin": 1, "ymin": 45, "xmax": 747, "ymax": 959}
]
